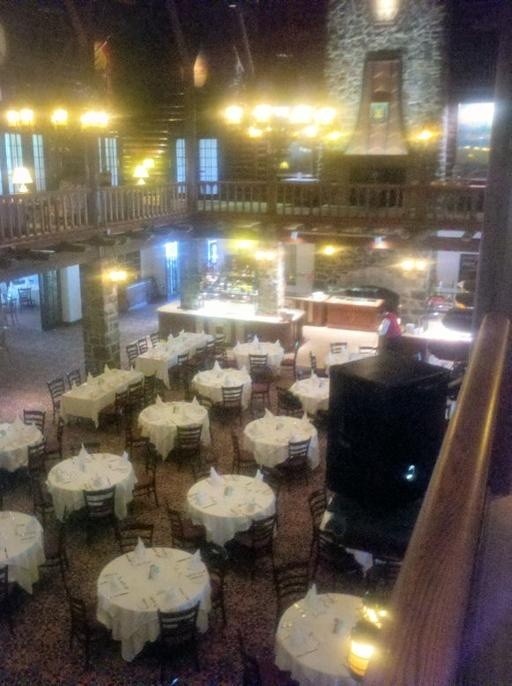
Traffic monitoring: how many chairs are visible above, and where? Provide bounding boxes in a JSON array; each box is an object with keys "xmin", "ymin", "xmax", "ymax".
[
  {"xmin": 125, "ymin": 323, "xmax": 382, "ymax": 436},
  {"xmin": 1, "ymin": 401, "xmax": 164, "ymax": 603},
  {"xmin": 64, "ymin": 522, "xmax": 231, "ymax": 683},
  {"xmin": 232, "ymin": 560, "xmax": 385, "ymax": 686},
  {"xmin": 0, "ymin": 278, "xmax": 37, "ymax": 323},
  {"xmin": 307, "ymin": 482, "xmax": 414, "ymax": 593},
  {"xmin": 47, "ymin": 363, "xmax": 321, "ymax": 500}
]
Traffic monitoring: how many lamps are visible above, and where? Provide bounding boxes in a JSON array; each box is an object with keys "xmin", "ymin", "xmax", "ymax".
[
  {"xmin": 12, "ymin": 167, "xmax": 33, "ymax": 193},
  {"xmin": 133, "ymin": 167, "xmax": 148, "ymax": 185}
]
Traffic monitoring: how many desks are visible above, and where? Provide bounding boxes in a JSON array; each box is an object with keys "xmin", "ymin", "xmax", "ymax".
[
  {"xmin": 325, "ymin": 295, "xmax": 386, "ymax": 332},
  {"xmin": 288, "ymin": 293, "xmax": 330, "ymax": 325}
]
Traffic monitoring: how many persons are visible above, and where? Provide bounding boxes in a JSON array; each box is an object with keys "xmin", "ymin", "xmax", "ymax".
[
  {"xmin": 442, "ymin": 292, "xmax": 473, "ymax": 333},
  {"xmin": 377, "ymin": 302, "xmax": 401, "ymax": 351}
]
